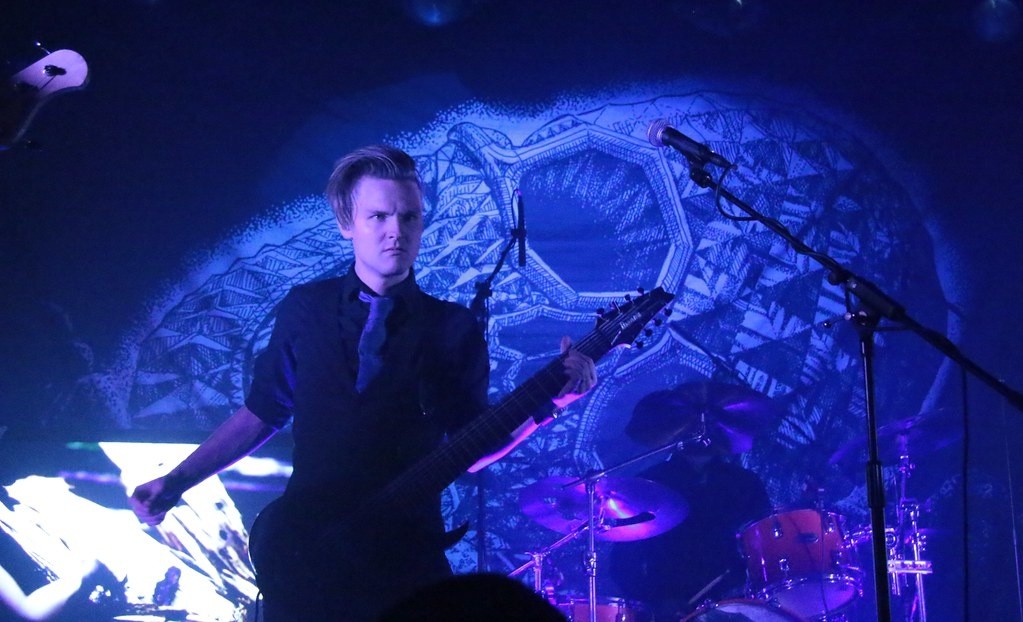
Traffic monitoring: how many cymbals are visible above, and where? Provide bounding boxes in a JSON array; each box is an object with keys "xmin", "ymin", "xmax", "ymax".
[
  {"xmin": 625, "ymin": 378, "xmax": 779, "ymax": 455},
  {"xmin": 519, "ymin": 469, "xmax": 692, "ymax": 544},
  {"xmin": 828, "ymin": 405, "xmax": 954, "ymax": 479}
]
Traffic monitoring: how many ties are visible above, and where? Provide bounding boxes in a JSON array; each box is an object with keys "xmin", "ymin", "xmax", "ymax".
[{"xmin": 354, "ymin": 292, "xmax": 395, "ymax": 395}]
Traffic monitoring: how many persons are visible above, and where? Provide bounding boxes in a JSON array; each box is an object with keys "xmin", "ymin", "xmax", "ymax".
[
  {"xmin": 611, "ymin": 448, "xmax": 772, "ymax": 622},
  {"xmin": 130, "ymin": 145, "xmax": 597, "ymax": 622}
]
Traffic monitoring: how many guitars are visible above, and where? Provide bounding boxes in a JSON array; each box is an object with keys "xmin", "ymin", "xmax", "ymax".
[{"xmin": 249, "ymin": 285, "xmax": 678, "ymax": 621}]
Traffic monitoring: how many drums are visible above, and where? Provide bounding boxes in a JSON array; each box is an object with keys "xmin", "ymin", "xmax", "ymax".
[
  {"xmin": 540, "ymin": 591, "xmax": 656, "ymax": 622},
  {"xmin": 674, "ymin": 597, "xmax": 807, "ymax": 621},
  {"xmin": 735, "ymin": 504, "xmax": 866, "ymax": 620}
]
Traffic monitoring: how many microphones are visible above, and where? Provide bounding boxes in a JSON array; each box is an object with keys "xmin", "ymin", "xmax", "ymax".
[
  {"xmin": 517, "ymin": 194, "xmax": 526, "ymax": 267},
  {"xmin": 645, "ymin": 116, "xmax": 732, "ymax": 171}
]
